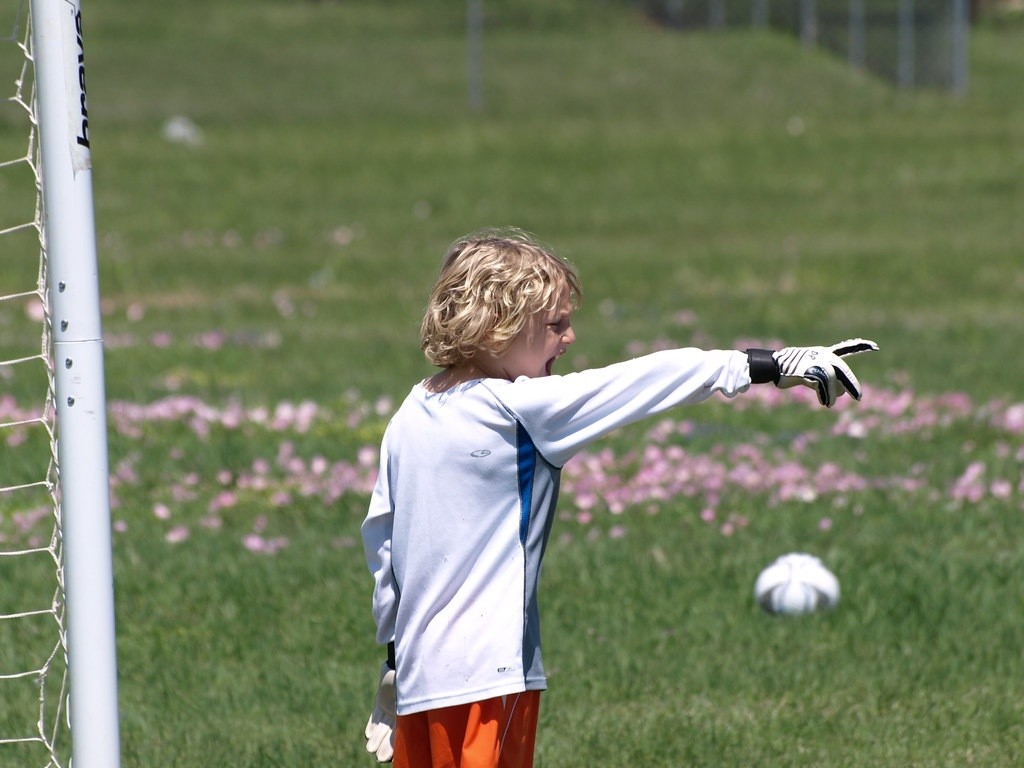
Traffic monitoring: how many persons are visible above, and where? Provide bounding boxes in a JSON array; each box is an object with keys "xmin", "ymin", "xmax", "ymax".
[{"xmin": 364, "ymin": 227, "xmax": 878, "ymax": 768}]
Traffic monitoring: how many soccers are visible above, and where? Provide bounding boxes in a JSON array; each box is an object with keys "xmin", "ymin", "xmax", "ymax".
[{"xmin": 753, "ymin": 550, "xmax": 843, "ymax": 622}]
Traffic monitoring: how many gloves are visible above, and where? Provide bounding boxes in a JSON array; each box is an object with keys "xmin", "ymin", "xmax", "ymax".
[
  {"xmin": 747, "ymin": 338, "xmax": 880, "ymax": 410},
  {"xmin": 363, "ymin": 660, "xmax": 396, "ymax": 763}
]
